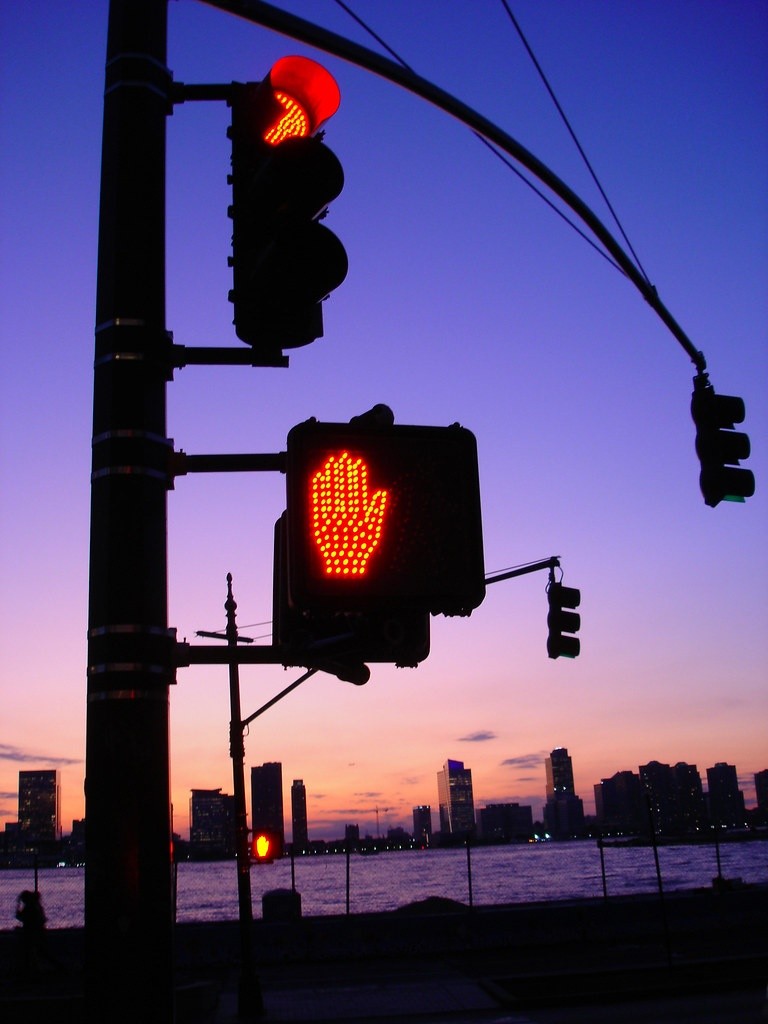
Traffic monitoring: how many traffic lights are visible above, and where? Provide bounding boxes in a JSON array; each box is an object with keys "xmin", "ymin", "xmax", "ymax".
[
  {"xmin": 688, "ymin": 373, "xmax": 757, "ymax": 509},
  {"xmin": 252, "ymin": 831, "xmax": 274, "ymax": 866},
  {"xmin": 546, "ymin": 584, "xmax": 583, "ymax": 663},
  {"xmin": 282, "ymin": 414, "xmax": 486, "ymax": 629},
  {"xmin": 220, "ymin": 56, "xmax": 349, "ymax": 354}
]
